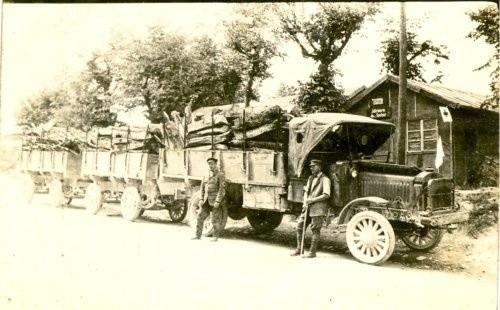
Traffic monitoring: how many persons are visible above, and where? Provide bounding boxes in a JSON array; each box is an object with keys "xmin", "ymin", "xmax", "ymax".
[
  {"xmin": 289, "ymin": 158, "xmax": 332, "ymax": 259},
  {"xmin": 188, "ymin": 157, "xmax": 227, "ymax": 242}
]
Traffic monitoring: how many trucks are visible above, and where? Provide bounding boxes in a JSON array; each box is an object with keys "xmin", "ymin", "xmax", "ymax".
[{"xmin": 19, "ymin": 100, "xmax": 470, "ymax": 265}]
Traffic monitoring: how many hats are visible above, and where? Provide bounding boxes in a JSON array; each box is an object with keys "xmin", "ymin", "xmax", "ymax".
[
  {"xmin": 309, "ymin": 160, "xmax": 323, "ymax": 167},
  {"xmin": 207, "ymin": 158, "xmax": 218, "ymax": 164}
]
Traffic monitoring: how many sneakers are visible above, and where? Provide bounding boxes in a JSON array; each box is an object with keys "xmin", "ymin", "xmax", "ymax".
[
  {"xmin": 289, "ymin": 249, "xmax": 301, "ymax": 256},
  {"xmin": 302, "ymin": 251, "xmax": 316, "ymax": 258}
]
